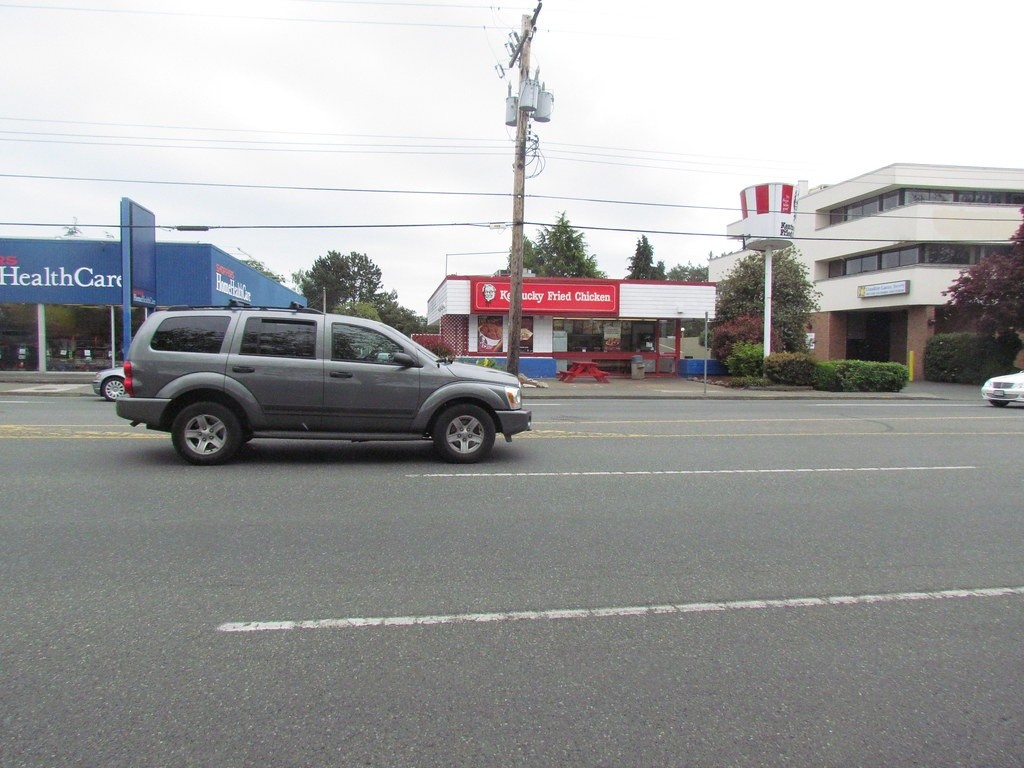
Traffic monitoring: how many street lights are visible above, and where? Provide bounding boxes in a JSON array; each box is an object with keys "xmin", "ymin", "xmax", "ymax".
[{"xmin": 681, "ymin": 327, "xmax": 685, "ymax": 360}]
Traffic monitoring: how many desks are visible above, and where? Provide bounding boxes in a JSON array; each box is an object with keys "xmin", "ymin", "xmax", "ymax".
[{"xmin": 571, "ymin": 361, "xmax": 599, "ymax": 374}]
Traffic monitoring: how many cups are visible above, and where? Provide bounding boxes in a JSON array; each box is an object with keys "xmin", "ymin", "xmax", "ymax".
[{"xmin": 478, "ymin": 324, "xmax": 502, "ymax": 351}]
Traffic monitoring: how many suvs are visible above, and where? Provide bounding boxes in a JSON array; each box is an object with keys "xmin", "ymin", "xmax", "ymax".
[{"xmin": 117, "ymin": 298, "xmax": 534, "ymax": 467}]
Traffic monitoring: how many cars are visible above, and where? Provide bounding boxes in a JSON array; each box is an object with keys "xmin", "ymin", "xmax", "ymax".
[
  {"xmin": 93, "ymin": 367, "xmax": 128, "ymax": 403},
  {"xmin": 981, "ymin": 369, "xmax": 1024, "ymax": 408}
]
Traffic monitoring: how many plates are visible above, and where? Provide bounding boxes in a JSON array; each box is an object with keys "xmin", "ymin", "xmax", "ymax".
[{"xmin": 606, "ymin": 341, "xmax": 619, "ymax": 347}]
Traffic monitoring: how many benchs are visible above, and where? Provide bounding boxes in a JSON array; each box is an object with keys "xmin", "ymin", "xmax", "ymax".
[
  {"xmin": 599, "ymin": 371, "xmax": 610, "ymax": 381},
  {"xmin": 560, "ymin": 370, "xmax": 575, "ymax": 383}
]
